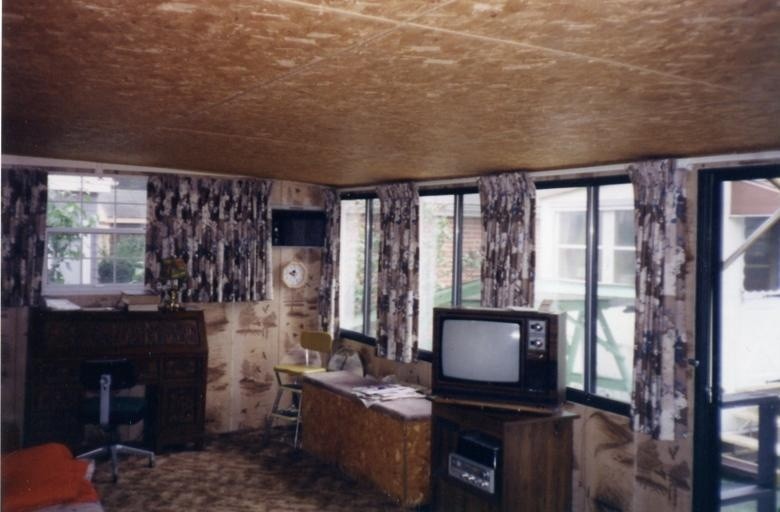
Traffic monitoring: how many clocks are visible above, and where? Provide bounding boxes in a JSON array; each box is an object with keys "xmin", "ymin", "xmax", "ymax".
[{"xmin": 281, "ymin": 261, "xmax": 308, "ymax": 289}]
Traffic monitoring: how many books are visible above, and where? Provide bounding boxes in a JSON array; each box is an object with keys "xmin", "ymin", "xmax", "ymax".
[{"xmin": 350, "ymin": 383, "xmax": 427, "ymax": 410}]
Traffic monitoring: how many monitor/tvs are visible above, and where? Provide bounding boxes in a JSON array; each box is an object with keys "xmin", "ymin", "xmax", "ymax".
[{"xmin": 432, "ymin": 304, "xmax": 567, "ymax": 406}]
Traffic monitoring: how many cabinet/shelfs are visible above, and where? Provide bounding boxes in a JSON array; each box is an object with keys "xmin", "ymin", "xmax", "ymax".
[
  {"xmin": 300, "ymin": 367, "xmax": 434, "ymax": 507},
  {"xmin": 22, "ymin": 304, "xmax": 208, "ymax": 452},
  {"xmin": 425, "ymin": 393, "xmax": 582, "ymax": 512}
]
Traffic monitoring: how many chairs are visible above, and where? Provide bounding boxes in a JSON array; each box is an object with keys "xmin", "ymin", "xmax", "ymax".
[
  {"xmin": 267, "ymin": 329, "xmax": 331, "ymax": 448},
  {"xmin": 0, "ymin": 441, "xmax": 106, "ymax": 512}
]
